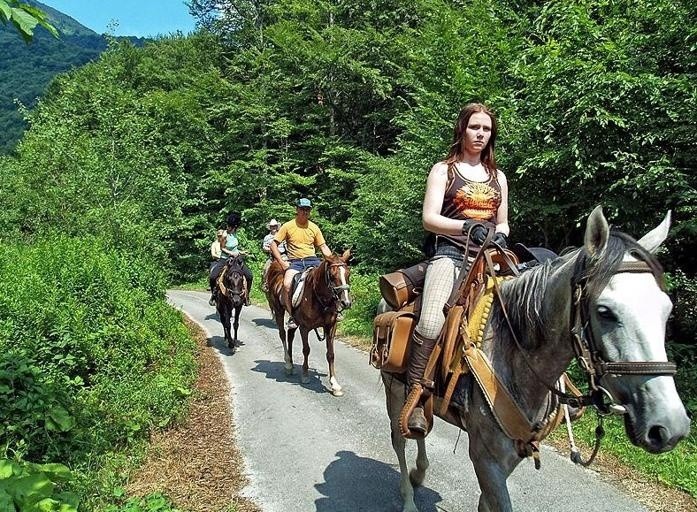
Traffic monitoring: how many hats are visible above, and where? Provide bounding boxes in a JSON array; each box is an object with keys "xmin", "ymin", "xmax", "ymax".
[
  {"xmin": 297, "ymin": 198, "xmax": 312, "ymax": 208},
  {"xmin": 266, "ymin": 218, "xmax": 281, "ymax": 231},
  {"xmin": 217, "ymin": 229, "xmax": 224, "ymax": 236}
]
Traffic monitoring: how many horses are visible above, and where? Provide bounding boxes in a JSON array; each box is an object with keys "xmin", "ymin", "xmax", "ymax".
[
  {"xmin": 216, "ymin": 255, "xmax": 244, "ymax": 349},
  {"xmin": 262, "ymin": 254, "xmax": 289, "ymax": 326},
  {"xmin": 267, "ymin": 248, "xmax": 352, "ymax": 397},
  {"xmin": 377, "ymin": 204, "xmax": 690, "ymax": 512}
]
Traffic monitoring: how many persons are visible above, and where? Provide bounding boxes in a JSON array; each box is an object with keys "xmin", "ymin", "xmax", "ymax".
[
  {"xmin": 206, "ymin": 229, "xmax": 223, "ymax": 290},
  {"xmin": 270, "ymin": 198, "xmax": 332, "ymax": 328},
  {"xmin": 209, "ymin": 215, "xmax": 252, "ymax": 307},
  {"xmin": 262, "ymin": 220, "xmax": 287, "ymax": 290},
  {"xmin": 406, "ymin": 100, "xmax": 509, "ymax": 432}
]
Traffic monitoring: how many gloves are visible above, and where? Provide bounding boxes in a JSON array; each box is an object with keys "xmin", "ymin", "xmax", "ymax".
[
  {"xmin": 491, "ymin": 232, "xmax": 509, "ymax": 248},
  {"xmin": 462, "ymin": 219, "xmax": 491, "ymax": 246}
]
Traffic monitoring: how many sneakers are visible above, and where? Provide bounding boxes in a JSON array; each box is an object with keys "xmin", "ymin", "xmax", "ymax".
[{"xmin": 287, "ymin": 319, "xmax": 297, "ymax": 329}]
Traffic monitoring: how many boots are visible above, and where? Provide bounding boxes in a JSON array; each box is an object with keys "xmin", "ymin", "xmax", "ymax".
[{"xmin": 403, "ymin": 330, "xmax": 438, "ymax": 432}]
